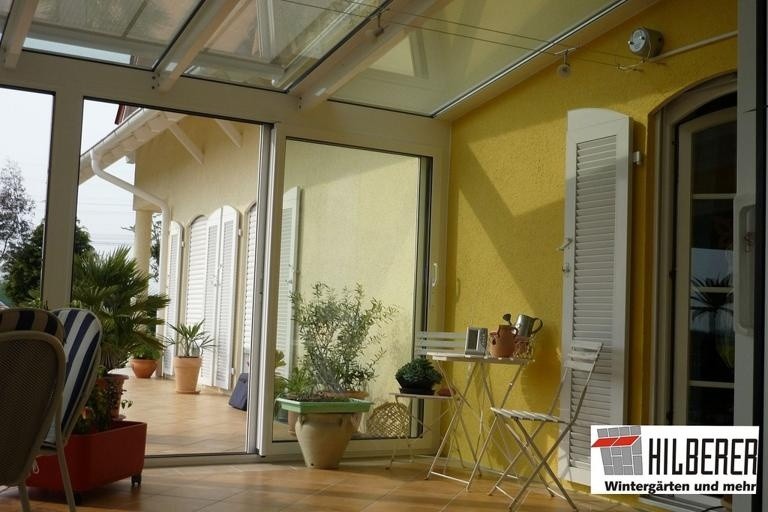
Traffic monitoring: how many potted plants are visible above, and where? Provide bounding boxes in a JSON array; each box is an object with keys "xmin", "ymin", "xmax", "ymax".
[
  {"xmin": 26, "ymin": 367, "xmax": 147, "ymax": 504},
  {"xmin": 160, "ymin": 318, "xmax": 217, "ymax": 394},
  {"xmin": 73, "ymin": 243, "xmax": 171, "ymax": 421},
  {"xmin": 275, "ymin": 281, "xmax": 401, "ymax": 469},
  {"xmin": 131, "ymin": 329, "xmax": 160, "ymax": 378},
  {"xmin": 394, "ymin": 355, "xmax": 441, "ymax": 395}
]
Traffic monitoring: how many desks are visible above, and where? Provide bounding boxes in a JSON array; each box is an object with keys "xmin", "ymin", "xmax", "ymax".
[{"xmin": 426, "ymin": 351, "xmax": 534, "ymax": 492}]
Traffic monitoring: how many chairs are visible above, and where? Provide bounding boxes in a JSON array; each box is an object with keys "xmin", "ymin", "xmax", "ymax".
[
  {"xmin": 50, "ymin": 306, "xmax": 103, "ymax": 457},
  {"xmin": 386, "ymin": 330, "xmax": 467, "ymax": 476},
  {"xmin": 488, "ymin": 339, "xmax": 605, "ymax": 512},
  {"xmin": 0, "ymin": 307, "xmax": 82, "ymax": 511}
]
